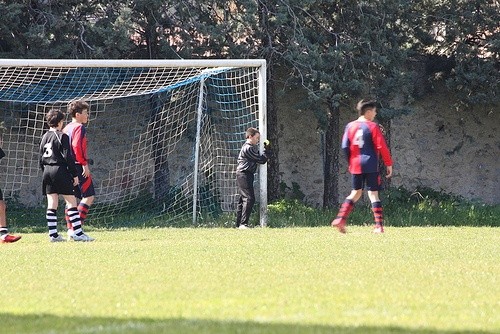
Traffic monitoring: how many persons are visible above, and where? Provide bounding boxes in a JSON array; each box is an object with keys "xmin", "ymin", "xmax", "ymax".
[
  {"xmin": 235, "ymin": 127, "xmax": 272, "ymax": 230},
  {"xmin": 0, "ymin": 148, "xmax": 21, "ymax": 244},
  {"xmin": 61, "ymin": 101, "xmax": 95, "ymax": 239},
  {"xmin": 331, "ymin": 98, "xmax": 392, "ymax": 234},
  {"xmin": 38, "ymin": 109, "xmax": 95, "ymax": 242}
]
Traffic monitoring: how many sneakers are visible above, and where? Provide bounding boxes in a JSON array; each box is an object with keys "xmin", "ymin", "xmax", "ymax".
[
  {"xmin": 50, "ymin": 234, "xmax": 67, "ymax": 242},
  {"xmin": 1, "ymin": 235, "xmax": 22, "ymax": 242},
  {"xmin": 73, "ymin": 233, "xmax": 93, "ymax": 241}
]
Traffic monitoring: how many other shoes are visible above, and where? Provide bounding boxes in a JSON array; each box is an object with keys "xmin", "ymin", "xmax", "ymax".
[
  {"xmin": 332, "ymin": 218, "xmax": 346, "ymax": 234},
  {"xmin": 373, "ymin": 226, "xmax": 384, "ymax": 233}
]
